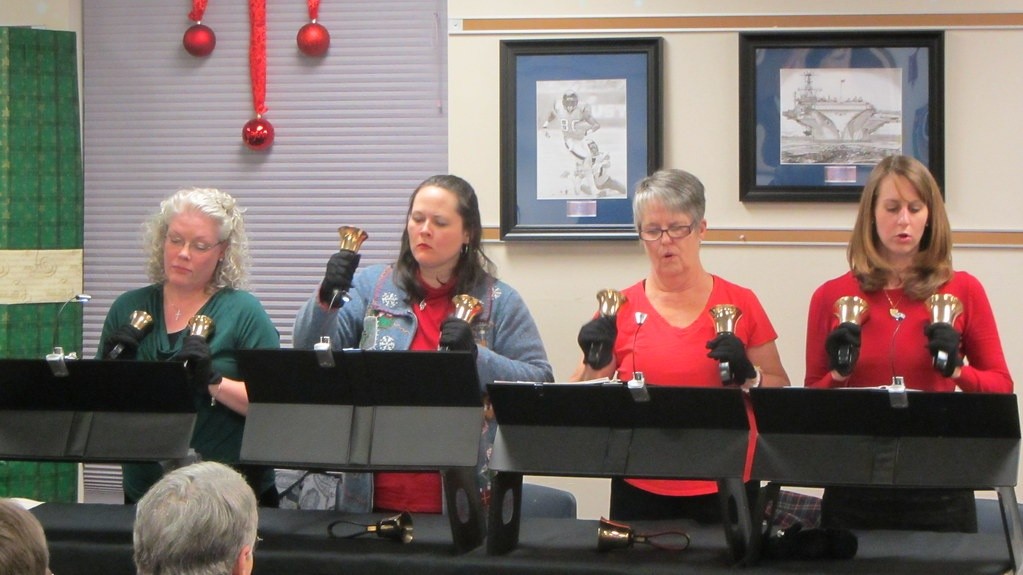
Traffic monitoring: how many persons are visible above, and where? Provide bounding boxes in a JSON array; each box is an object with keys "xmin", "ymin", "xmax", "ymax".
[
  {"xmin": 93, "ymin": 185, "xmax": 279, "ymax": 509},
  {"xmin": 291, "ymin": 174, "xmax": 554, "ymax": 514},
  {"xmin": 805, "ymin": 152, "xmax": 1015, "ymax": 533},
  {"xmin": 132, "ymin": 461, "xmax": 257, "ymax": 575},
  {"xmin": 568, "ymin": 166, "xmax": 792, "ymax": 521},
  {"xmin": 0, "ymin": 496, "xmax": 52, "ymax": 575},
  {"xmin": 543, "ymin": 87, "xmax": 624, "ymax": 198}
]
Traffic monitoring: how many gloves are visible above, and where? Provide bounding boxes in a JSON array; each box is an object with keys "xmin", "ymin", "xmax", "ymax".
[
  {"xmin": 578, "ymin": 315, "xmax": 617, "ymax": 371},
  {"xmin": 438, "ymin": 315, "xmax": 476, "ymax": 351},
  {"xmin": 321, "ymin": 250, "xmax": 360, "ymax": 308},
  {"xmin": 825, "ymin": 320, "xmax": 862, "ymax": 376},
  {"xmin": 924, "ymin": 323, "xmax": 958, "ymax": 376},
  {"xmin": 706, "ymin": 335, "xmax": 757, "ymax": 387}
]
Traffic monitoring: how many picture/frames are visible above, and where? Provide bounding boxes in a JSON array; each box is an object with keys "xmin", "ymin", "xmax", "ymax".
[
  {"xmin": 735, "ymin": 29, "xmax": 946, "ymax": 202},
  {"xmin": 497, "ymin": 38, "xmax": 663, "ymax": 240}
]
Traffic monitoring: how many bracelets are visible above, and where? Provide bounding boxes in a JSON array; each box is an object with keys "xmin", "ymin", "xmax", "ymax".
[
  {"xmin": 211, "ymin": 377, "xmax": 225, "ymax": 406},
  {"xmin": 742, "ymin": 366, "xmax": 762, "ymax": 396}
]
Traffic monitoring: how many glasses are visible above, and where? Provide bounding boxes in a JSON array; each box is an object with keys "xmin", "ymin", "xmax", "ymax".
[
  {"xmin": 165, "ymin": 231, "xmax": 225, "ymax": 255},
  {"xmin": 639, "ymin": 219, "xmax": 698, "ymax": 242}
]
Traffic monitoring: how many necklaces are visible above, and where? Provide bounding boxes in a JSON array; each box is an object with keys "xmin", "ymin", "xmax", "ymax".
[
  {"xmin": 883, "ymin": 286, "xmax": 906, "ymax": 321},
  {"xmin": 419, "ymin": 299, "xmax": 427, "ymax": 311},
  {"xmin": 168, "ymin": 300, "xmax": 182, "ymax": 321}
]
